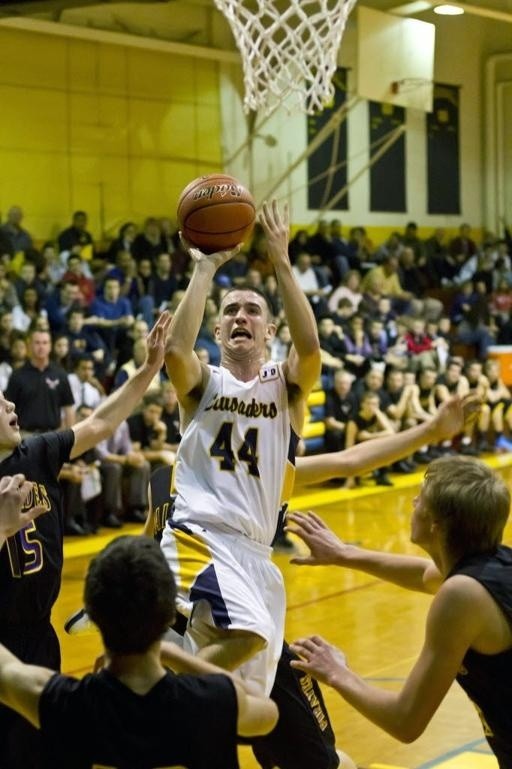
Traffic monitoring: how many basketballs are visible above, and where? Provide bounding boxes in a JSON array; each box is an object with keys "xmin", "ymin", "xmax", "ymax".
[{"xmin": 177, "ymin": 174, "xmax": 254, "ymax": 251}]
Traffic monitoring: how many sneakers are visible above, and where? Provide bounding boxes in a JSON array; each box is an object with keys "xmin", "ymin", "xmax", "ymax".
[
  {"xmin": 64, "ymin": 509, "xmax": 146, "ymax": 536},
  {"xmin": 375, "ymin": 436, "xmax": 512, "ymax": 486}
]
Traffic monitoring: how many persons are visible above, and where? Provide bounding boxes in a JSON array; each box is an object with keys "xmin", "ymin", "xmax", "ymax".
[
  {"xmin": 141, "ymin": 388, "xmax": 485, "ymax": 769},
  {"xmin": 281, "ymin": 454, "xmax": 512, "ymax": 769},
  {"xmin": 0, "ymin": 472, "xmax": 281, "ymax": 769},
  {"xmin": 1, "ymin": 309, "xmax": 176, "ymax": 674},
  {"xmin": 0, "ymin": 205, "xmax": 512, "ymax": 535},
  {"xmin": 65, "ymin": 195, "xmax": 322, "ymax": 698}
]
